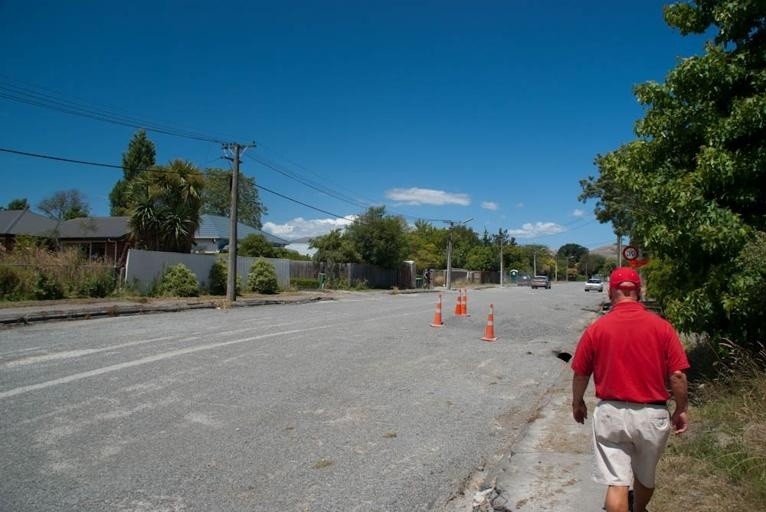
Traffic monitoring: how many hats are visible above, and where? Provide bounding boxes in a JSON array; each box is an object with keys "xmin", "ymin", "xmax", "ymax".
[{"xmin": 607, "ymin": 266, "xmax": 641, "ymax": 292}]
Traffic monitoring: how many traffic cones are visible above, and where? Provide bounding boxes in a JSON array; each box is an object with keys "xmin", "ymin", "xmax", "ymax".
[
  {"xmin": 452, "ymin": 287, "xmax": 471, "ymax": 317},
  {"xmin": 429, "ymin": 293, "xmax": 446, "ymax": 327},
  {"xmin": 480, "ymin": 303, "xmax": 498, "ymax": 342}
]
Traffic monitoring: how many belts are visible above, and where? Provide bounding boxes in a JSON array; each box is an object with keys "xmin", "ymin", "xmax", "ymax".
[{"xmin": 600, "ymin": 397, "xmax": 668, "ymax": 406}]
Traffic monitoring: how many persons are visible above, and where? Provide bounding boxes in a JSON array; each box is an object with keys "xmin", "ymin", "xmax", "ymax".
[{"xmin": 570, "ymin": 266, "xmax": 692, "ymax": 511}]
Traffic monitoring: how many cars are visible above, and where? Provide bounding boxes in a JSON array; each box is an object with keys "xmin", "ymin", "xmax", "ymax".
[
  {"xmin": 584, "ymin": 278, "xmax": 604, "ymax": 292},
  {"xmin": 516, "ymin": 273, "xmax": 552, "ymax": 289}
]
